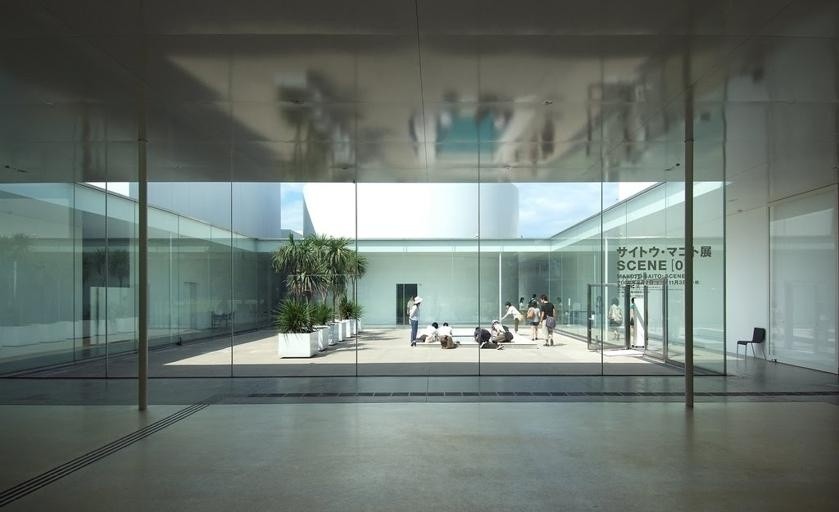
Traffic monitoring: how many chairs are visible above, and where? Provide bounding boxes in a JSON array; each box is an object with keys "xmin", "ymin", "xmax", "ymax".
[{"xmin": 736, "ymin": 327, "xmax": 766, "ymax": 360}]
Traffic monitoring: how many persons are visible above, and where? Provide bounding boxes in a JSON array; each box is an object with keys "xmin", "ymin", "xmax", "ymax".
[
  {"xmin": 407, "ymin": 295, "xmax": 414, "ymax": 315},
  {"xmin": 500, "ymin": 293, "xmax": 558, "ymax": 346},
  {"xmin": 439, "ymin": 321, "xmax": 453, "ymax": 349},
  {"xmin": 418, "ymin": 321, "xmax": 439, "ymax": 344},
  {"xmin": 409, "ymin": 296, "xmax": 424, "ymax": 347},
  {"xmin": 609, "ymin": 297, "xmax": 623, "ymax": 341},
  {"xmin": 491, "ymin": 320, "xmax": 508, "ymax": 350},
  {"xmin": 474, "ymin": 327, "xmax": 492, "ymax": 348},
  {"xmin": 630, "ymin": 296, "xmax": 637, "ymax": 336}
]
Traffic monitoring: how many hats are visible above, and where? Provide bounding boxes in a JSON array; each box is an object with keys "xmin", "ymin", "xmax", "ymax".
[{"xmin": 413, "ymin": 297, "xmax": 422, "ymax": 304}]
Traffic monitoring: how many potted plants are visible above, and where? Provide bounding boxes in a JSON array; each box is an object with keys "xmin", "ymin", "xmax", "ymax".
[{"xmin": 271, "ymin": 233, "xmax": 369, "ymax": 358}]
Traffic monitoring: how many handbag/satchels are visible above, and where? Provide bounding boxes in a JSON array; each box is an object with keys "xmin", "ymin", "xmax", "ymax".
[
  {"xmin": 527, "ymin": 309, "xmax": 535, "ymax": 318},
  {"xmin": 546, "ymin": 318, "xmax": 556, "ymax": 329}
]
